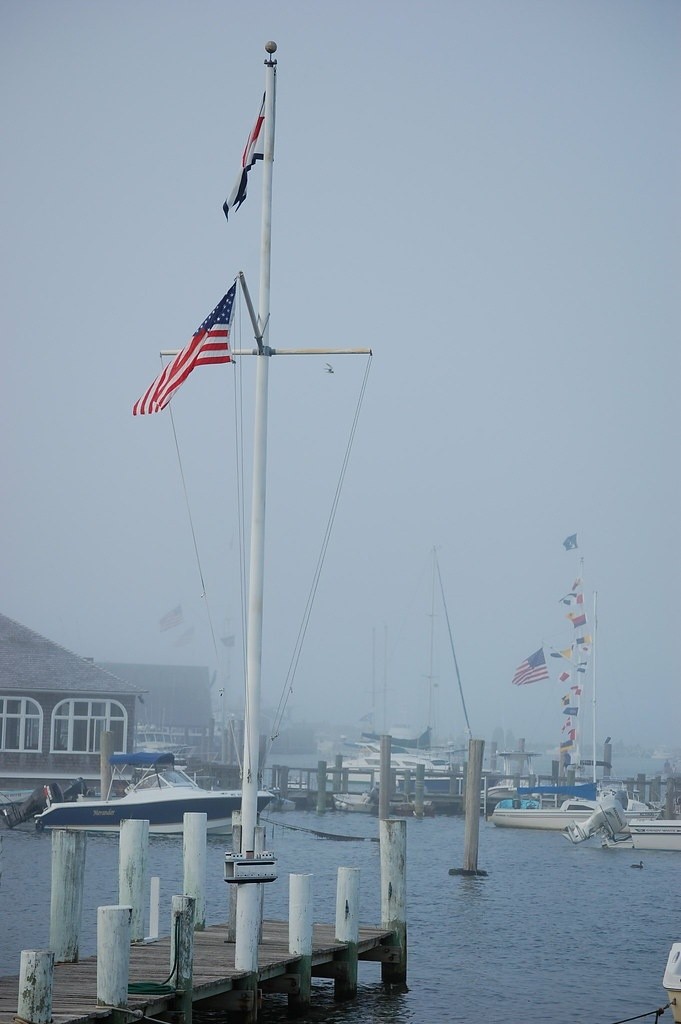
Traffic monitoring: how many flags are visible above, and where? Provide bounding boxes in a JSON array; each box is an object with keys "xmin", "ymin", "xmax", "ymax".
[
  {"xmin": 222, "ymin": 90, "xmax": 267, "ymax": 220},
  {"xmin": 550, "ymin": 575, "xmax": 591, "ymax": 752},
  {"xmin": 133, "ymin": 282, "xmax": 237, "ymax": 417},
  {"xmin": 512, "ymin": 649, "xmax": 550, "ymax": 686},
  {"xmin": 563, "ymin": 533, "xmax": 578, "ymax": 550}
]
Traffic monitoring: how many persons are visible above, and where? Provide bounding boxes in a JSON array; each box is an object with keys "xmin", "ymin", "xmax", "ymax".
[{"xmin": 563, "ymin": 751, "xmax": 571, "ymax": 777}]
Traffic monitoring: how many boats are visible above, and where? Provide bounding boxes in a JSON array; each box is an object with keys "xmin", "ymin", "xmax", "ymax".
[
  {"xmin": 33, "ymin": 752, "xmax": 274, "ymax": 836},
  {"xmin": 628, "ymin": 818, "xmax": 681, "ymax": 847},
  {"xmin": 333, "ymin": 789, "xmax": 434, "ymax": 816},
  {"xmin": 485, "ymin": 797, "xmax": 663, "ymax": 831},
  {"xmin": 651, "ymin": 745, "xmax": 675, "ymax": 760},
  {"xmin": 324, "ymin": 741, "xmax": 502, "ymax": 793},
  {"xmin": 338, "ymin": 732, "xmax": 348, "ymax": 740}
]
